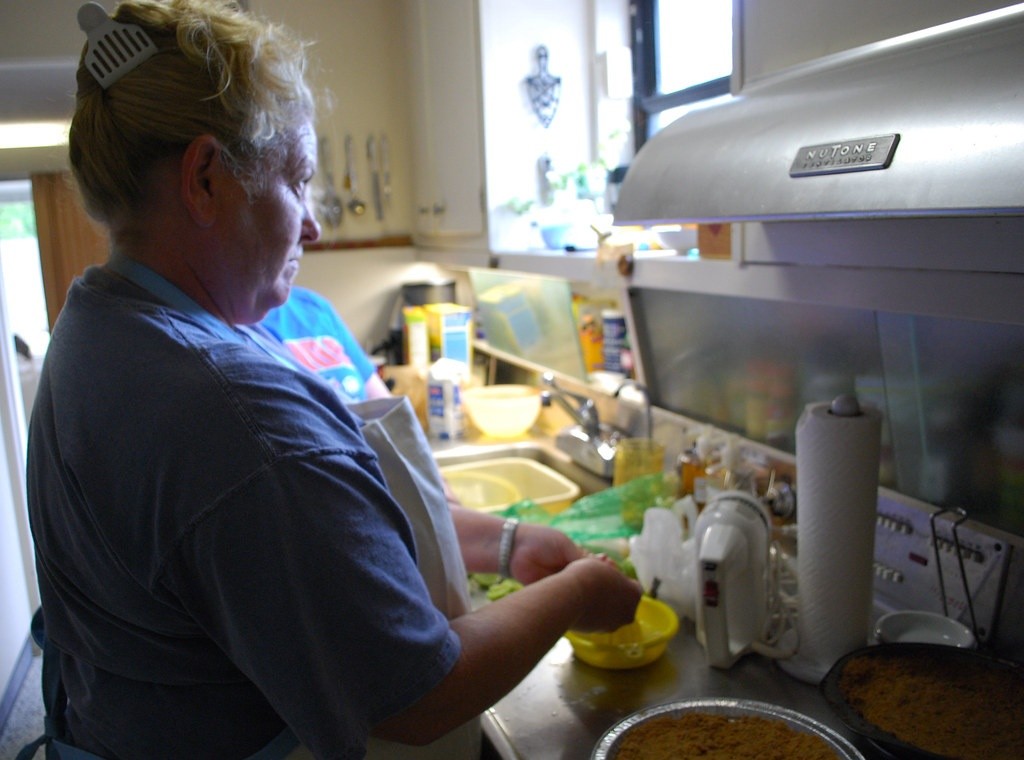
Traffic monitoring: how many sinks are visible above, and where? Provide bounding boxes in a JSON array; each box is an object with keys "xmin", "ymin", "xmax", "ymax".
[{"xmin": 435, "ymin": 440, "xmax": 615, "ymax": 524}]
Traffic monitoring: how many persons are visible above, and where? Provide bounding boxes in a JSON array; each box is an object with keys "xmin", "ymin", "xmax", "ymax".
[
  {"xmin": 15, "ymin": 335, "xmax": 40, "ymax": 427},
  {"xmin": 26, "ymin": 1, "xmax": 644, "ymax": 760}
]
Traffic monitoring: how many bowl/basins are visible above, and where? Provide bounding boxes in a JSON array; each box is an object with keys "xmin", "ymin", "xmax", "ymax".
[
  {"xmin": 540, "ymin": 223, "xmax": 573, "ymax": 249},
  {"xmin": 565, "ymin": 595, "xmax": 679, "ymax": 670},
  {"xmin": 442, "ymin": 470, "xmax": 523, "ymax": 509},
  {"xmin": 657, "ymin": 230, "xmax": 698, "ymax": 256},
  {"xmin": 819, "ymin": 643, "xmax": 1024, "ymax": 760},
  {"xmin": 460, "ymin": 383, "xmax": 543, "ymax": 437},
  {"xmin": 874, "ymin": 610, "xmax": 976, "ymax": 649}
]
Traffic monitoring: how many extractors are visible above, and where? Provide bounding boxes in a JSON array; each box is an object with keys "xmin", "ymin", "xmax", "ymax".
[{"xmin": 612, "ymin": 0, "xmax": 1024, "ymax": 227}]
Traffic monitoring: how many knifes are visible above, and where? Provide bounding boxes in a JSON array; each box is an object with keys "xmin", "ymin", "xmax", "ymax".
[{"xmin": 367, "ymin": 135, "xmax": 392, "ymax": 221}]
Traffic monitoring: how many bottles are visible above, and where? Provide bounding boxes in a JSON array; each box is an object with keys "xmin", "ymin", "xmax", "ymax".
[{"xmin": 680, "ymin": 424, "xmax": 754, "ymax": 514}]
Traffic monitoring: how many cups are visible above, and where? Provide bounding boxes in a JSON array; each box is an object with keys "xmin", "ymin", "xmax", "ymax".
[{"xmin": 611, "ymin": 437, "xmax": 665, "ymax": 489}]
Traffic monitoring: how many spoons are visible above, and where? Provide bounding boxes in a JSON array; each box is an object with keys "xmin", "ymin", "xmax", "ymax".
[{"xmin": 345, "ymin": 132, "xmax": 367, "ymax": 215}]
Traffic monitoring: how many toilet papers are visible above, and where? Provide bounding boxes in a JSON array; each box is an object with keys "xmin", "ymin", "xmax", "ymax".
[{"xmin": 794, "ymin": 401, "xmax": 883, "ymax": 665}]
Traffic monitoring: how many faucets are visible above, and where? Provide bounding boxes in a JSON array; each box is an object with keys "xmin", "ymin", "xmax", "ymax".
[{"xmin": 540, "ymin": 373, "xmax": 602, "ymax": 439}]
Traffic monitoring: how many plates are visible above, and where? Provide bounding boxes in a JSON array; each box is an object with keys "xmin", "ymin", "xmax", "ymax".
[{"xmin": 589, "ymin": 697, "xmax": 866, "ymax": 760}]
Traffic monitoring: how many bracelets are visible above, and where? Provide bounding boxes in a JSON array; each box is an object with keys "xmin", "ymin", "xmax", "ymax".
[{"xmin": 499, "ymin": 518, "xmax": 519, "ymax": 577}]
